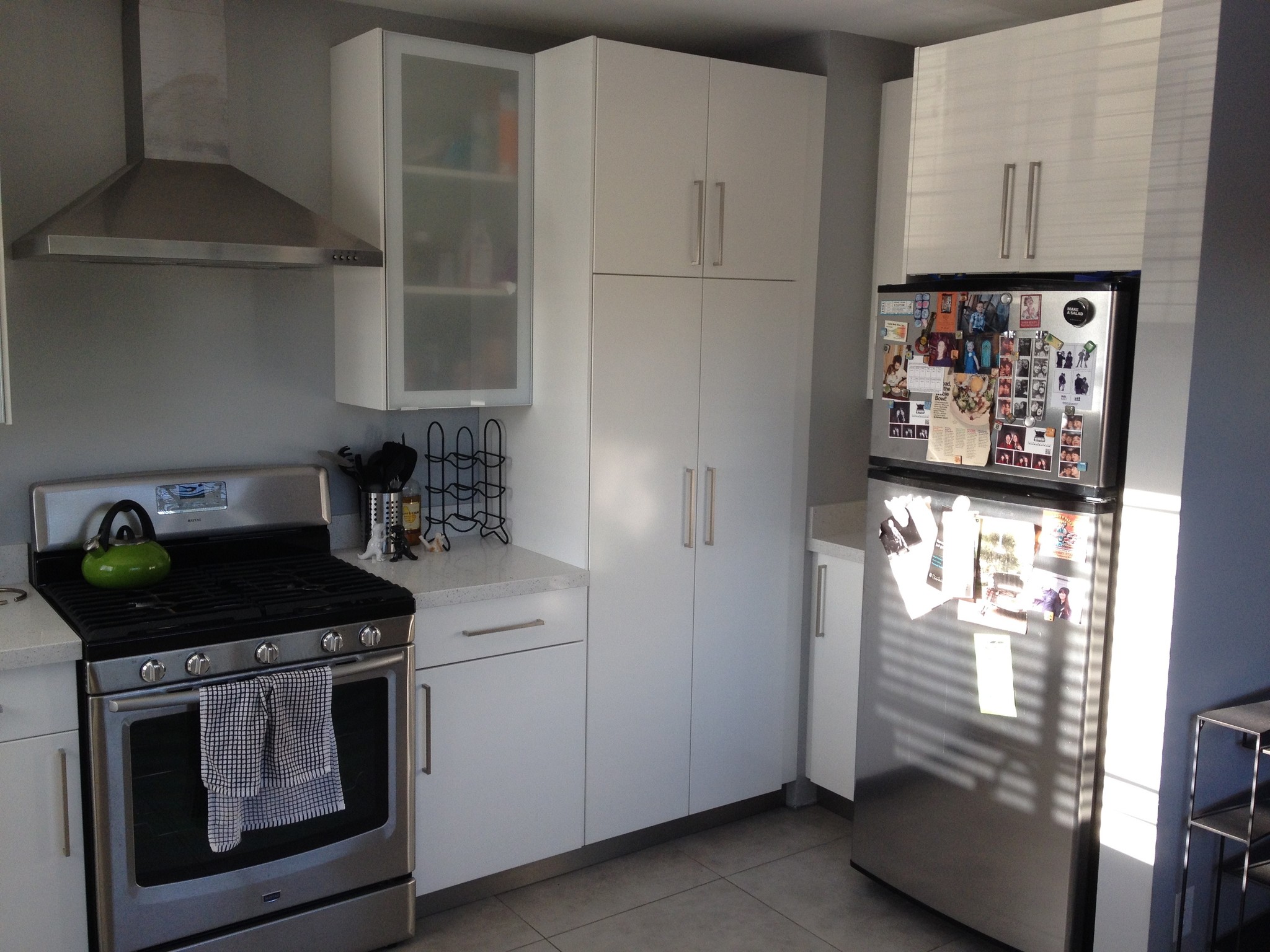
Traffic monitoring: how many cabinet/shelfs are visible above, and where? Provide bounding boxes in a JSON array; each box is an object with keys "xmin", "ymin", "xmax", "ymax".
[
  {"xmin": 327, "ymin": 26, "xmax": 536, "ymax": 411},
  {"xmin": 905, "ymin": 0, "xmax": 1164, "ymax": 277},
  {"xmin": 805, "ymin": 498, "xmax": 870, "ymax": 822},
  {"xmin": 0, "ymin": 541, "xmax": 91, "ymax": 952},
  {"xmin": 1172, "ymin": 699, "xmax": 1270, "ymax": 952},
  {"xmin": 331, "ymin": 535, "xmax": 589, "ymax": 914},
  {"xmin": 481, "ymin": 34, "xmax": 828, "ymax": 868}
]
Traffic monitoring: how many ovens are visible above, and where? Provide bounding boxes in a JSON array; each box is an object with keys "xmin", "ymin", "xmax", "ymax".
[{"xmin": 70, "ymin": 642, "xmax": 423, "ymax": 950}]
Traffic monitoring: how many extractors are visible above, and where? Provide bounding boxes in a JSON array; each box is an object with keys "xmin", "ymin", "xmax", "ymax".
[{"xmin": 1, "ymin": 2, "xmax": 387, "ymax": 271}]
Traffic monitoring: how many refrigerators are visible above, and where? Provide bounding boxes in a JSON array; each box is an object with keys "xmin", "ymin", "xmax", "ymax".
[{"xmin": 836, "ymin": 265, "xmax": 1143, "ymax": 951}]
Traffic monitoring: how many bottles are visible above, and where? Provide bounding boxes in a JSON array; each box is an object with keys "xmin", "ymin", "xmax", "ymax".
[{"xmin": 398, "ymin": 475, "xmax": 422, "ymax": 547}]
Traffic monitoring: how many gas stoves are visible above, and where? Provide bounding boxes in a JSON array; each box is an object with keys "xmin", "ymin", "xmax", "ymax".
[{"xmin": 22, "ymin": 458, "xmax": 418, "ymax": 695}]
